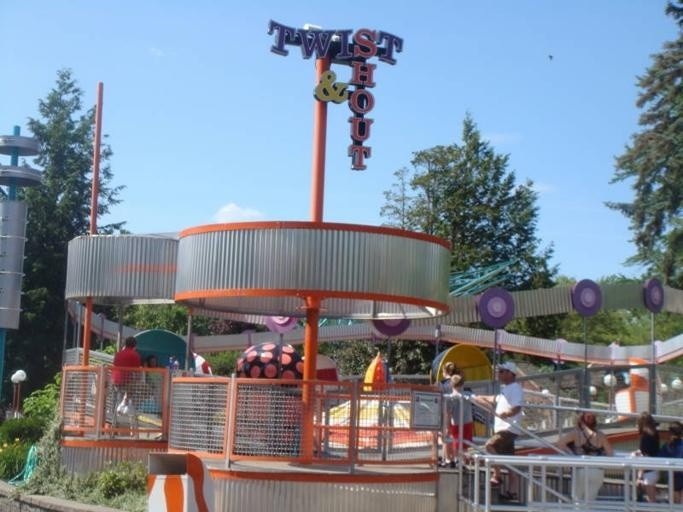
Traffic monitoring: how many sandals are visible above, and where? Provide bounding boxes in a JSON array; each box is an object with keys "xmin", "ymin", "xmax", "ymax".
[
  {"xmin": 501, "ymin": 492, "xmax": 521, "ymax": 503},
  {"xmin": 490, "ymin": 477, "xmax": 503, "ymax": 485},
  {"xmin": 438, "ymin": 460, "xmax": 457, "ymax": 468}
]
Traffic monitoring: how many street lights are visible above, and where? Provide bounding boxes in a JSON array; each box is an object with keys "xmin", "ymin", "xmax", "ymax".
[
  {"xmin": 15, "ymin": 369, "xmax": 27, "ymax": 421},
  {"xmin": 540, "ymin": 371, "xmax": 682, "ymax": 422},
  {"xmin": 10, "ymin": 374, "xmax": 19, "ymax": 417}
]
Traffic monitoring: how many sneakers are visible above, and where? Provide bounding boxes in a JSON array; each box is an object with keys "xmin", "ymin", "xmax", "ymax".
[{"xmin": 117, "ymin": 404, "xmax": 138, "ymax": 414}]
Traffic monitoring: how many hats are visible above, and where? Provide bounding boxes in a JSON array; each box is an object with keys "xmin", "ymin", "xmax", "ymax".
[{"xmin": 498, "ymin": 360, "xmax": 518, "ymax": 376}]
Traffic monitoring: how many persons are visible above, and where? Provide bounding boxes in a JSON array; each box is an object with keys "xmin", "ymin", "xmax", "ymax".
[
  {"xmin": 658, "ymin": 419, "xmax": 683, "ymax": 504},
  {"xmin": 559, "ymin": 412, "xmax": 613, "ymax": 504},
  {"xmin": 631, "ymin": 412, "xmax": 661, "ymax": 502},
  {"xmin": 434, "ymin": 361, "xmax": 465, "ymax": 470},
  {"xmin": 111, "ymin": 335, "xmax": 164, "ymax": 418},
  {"xmin": 442, "ymin": 372, "xmax": 494, "ymax": 471},
  {"xmin": 474, "ymin": 360, "xmax": 524, "ymax": 500}
]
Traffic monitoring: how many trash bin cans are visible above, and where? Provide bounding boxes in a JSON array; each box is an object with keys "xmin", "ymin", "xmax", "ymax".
[{"xmin": 147, "ymin": 453, "xmax": 216, "ymax": 512}]
